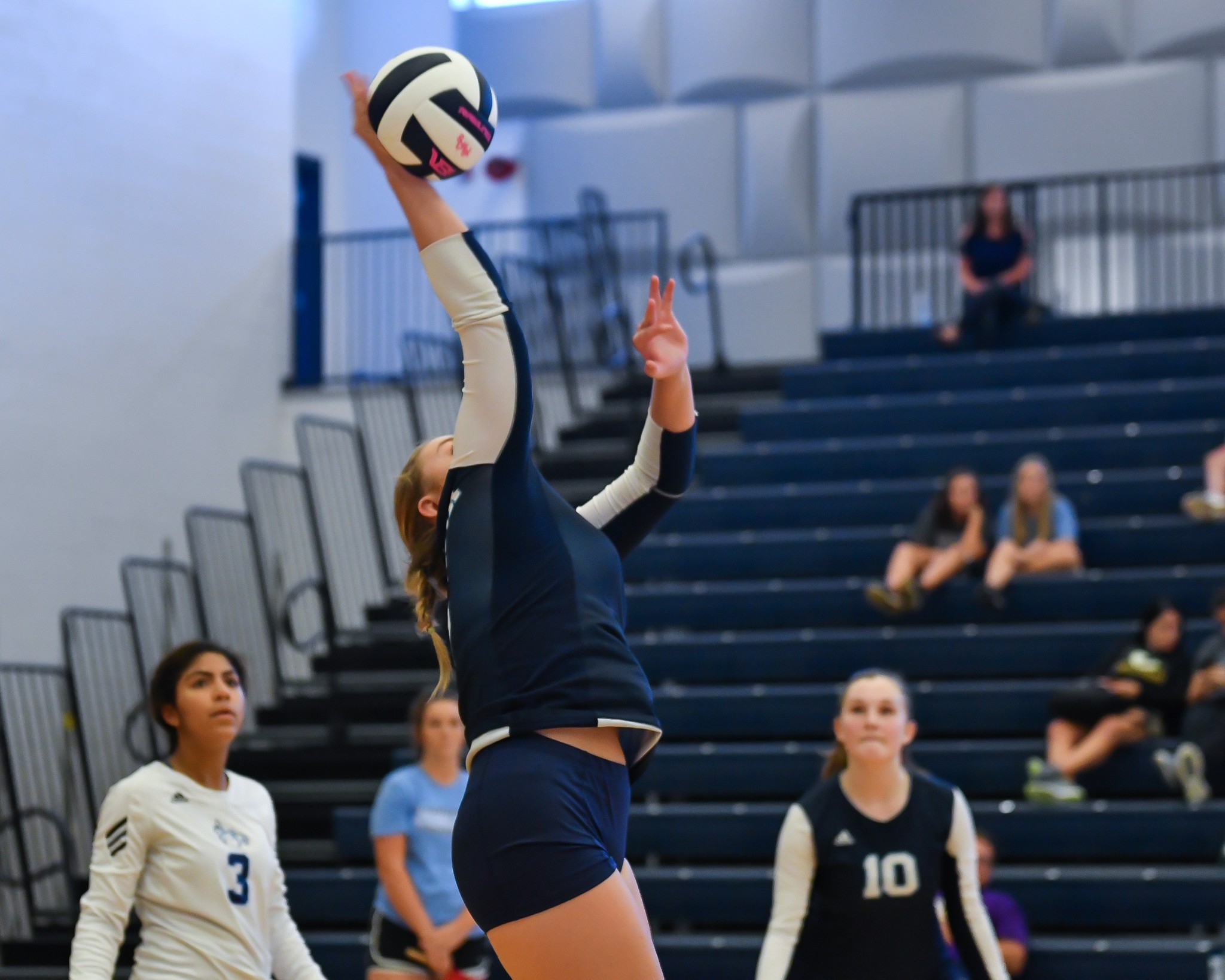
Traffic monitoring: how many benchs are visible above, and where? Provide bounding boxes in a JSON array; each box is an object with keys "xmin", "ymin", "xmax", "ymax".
[{"xmin": 0, "ymin": 296, "xmax": 1225, "ymax": 980}]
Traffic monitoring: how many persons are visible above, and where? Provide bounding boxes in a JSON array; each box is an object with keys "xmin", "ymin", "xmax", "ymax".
[
  {"xmin": 345, "ymin": 68, "xmax": 705, "ymax": 980},
  {"xmin": 939, "ymin": 187, "xmax": 1036, "ymax": 350},
  {"xmin": 68, "ymin": 449, "xmax": 1224, "ymax": 980}
]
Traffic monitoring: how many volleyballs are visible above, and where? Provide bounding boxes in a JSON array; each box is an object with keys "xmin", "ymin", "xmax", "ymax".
[{"xmin": 365, "ymin": 45, "xmax": 498, "ymax": 181}]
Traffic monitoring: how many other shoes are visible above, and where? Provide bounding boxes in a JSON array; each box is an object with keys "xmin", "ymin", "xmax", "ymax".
[
  {"xmin": 1153, "ymin": 746, "xmax": 1182, "ymax": 793},
  {"xmin": 865, "ymin": 582, "xmax": 899, "ymax": 611},
  {"xmin": 895, "ymin": 579, "xmax": 919, "ymax": 610},
  {"xmin": 1176, "ymin": 742, "xmax": 1208, "ymax": 804},
  {"xmin": 1025, "ymin": 754, "xmax": 1057, "ymax": 784},
  {"xmin": 1024, "ymin": 782, "xmax": 1086, "ymax": 801}
]
